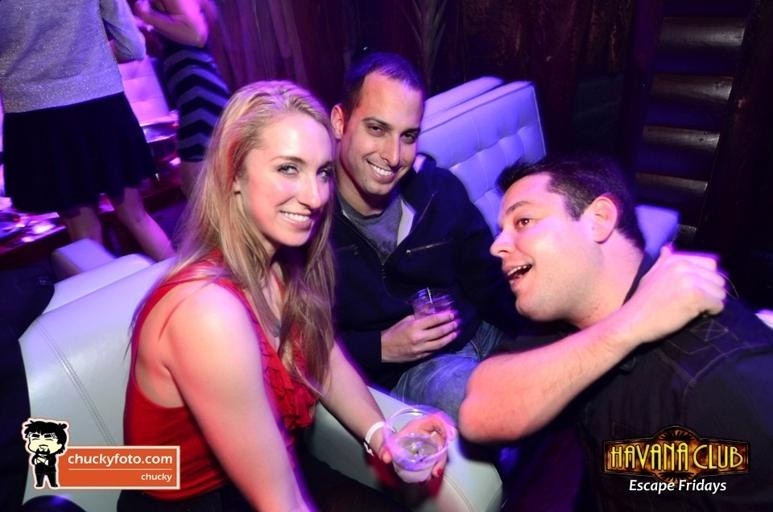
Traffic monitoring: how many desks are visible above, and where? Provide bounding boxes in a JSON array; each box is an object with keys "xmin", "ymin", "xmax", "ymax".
[{"xmin": 1, "ymin": 159, "xmax": 188, "ymax": 268}]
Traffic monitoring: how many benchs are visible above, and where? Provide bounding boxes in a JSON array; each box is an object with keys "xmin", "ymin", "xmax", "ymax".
[
  {"xmin": 405, "ymin": 75, "xmax": 502, "ymax": 118},
  {"xmin": 19, "ymin": 251, "xmax": 502, "ymax": 511},
  {"xmin": 31, "ymin": 238, "xmax": 155, "ymax": 315},
  {"xmin": 419, "ymin": 81, "xmax": 680, "ymax": 282},
  {"xmin": 1, "ymin": 56, "xmax": 170, "ymax": 193}
]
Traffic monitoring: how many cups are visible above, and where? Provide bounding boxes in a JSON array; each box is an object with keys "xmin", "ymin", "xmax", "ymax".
[
  {"xmin": 409, "ymin": 287, "xmax": 457, "ymax": 320},
  {"xmin": 382, "ymin": 406, "xmax": 452, "ymax": 484}
]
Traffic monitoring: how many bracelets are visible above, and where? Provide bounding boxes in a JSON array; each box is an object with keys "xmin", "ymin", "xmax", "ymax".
[{"xmin": 362, "ymin": 421, "xmax": 398, "ymax": 457}]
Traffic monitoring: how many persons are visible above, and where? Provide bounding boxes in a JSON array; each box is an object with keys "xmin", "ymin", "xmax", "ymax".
[
  {"xmin": 113, "ymin": 80, "xmax": 449, "ymax": 512},
  {"xmin": 0, "ymin": 0, "xmax": 176, "ymax": 263},
  {"xmin": 320, "ymin": 49, "xmax": 538, "ymax": 481},
  {"xmin": 456, "ymin": 156, "xmax": 773, "ymax": 512},
  {"xmin": 129, "ymin": 0, "xmax": 230, "ymax": 202}
]
